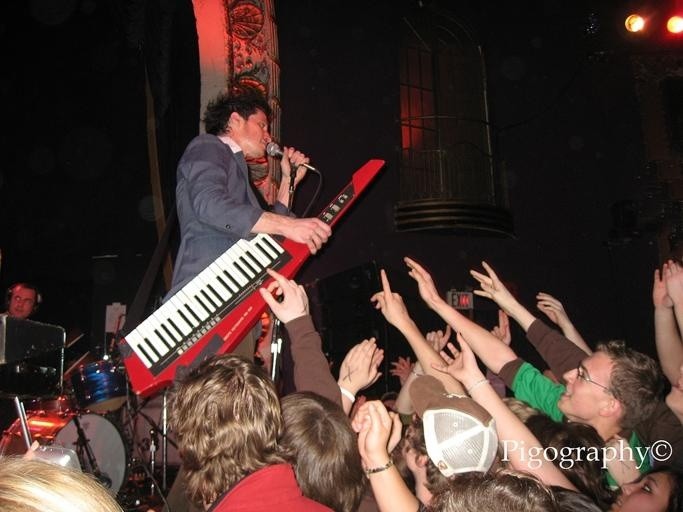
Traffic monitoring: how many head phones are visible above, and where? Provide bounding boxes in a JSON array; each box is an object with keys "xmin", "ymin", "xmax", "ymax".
[{"xmin": 5, "ymin": 281, "xmax": 42, "ymax": 313}]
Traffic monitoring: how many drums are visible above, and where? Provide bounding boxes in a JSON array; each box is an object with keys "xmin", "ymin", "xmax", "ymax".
[
  {"xmin": 68, "ymin": 361, "xmax": 127, "ymax": 414},
  {"xmin": 0, "ymin": 409, "xmax": 131, "ymax": 498}
]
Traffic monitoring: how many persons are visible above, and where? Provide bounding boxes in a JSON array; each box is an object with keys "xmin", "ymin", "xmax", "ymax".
[
  {"xmin": 159, "ymin": 84, "xmax": 332, "ymax": 364},
  {"xmin": 0, "ymin": 256, "xmax": 683, "ymax": 512}
]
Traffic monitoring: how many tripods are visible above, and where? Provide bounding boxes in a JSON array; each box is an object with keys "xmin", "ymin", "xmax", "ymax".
[{"xmin": 124, "ymin": 387, "xmax": 170, "ymax": 512}]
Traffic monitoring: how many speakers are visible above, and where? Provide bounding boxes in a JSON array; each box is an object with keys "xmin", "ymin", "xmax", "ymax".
[
  {"xmin": 316, "ymin": 261, "xmax": 405, "ymax": 401},
  {"xmin": 0, "ymin": 314, "xmax": 66, "ymax": 397}
]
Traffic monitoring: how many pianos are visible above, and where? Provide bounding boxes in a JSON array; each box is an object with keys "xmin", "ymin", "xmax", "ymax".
[{"xmin": 117, "ymin": 160, "xmax": 385, "ymax": 393}]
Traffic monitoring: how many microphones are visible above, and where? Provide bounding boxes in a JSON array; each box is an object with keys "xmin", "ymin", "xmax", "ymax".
[{"xmin": 266, "ymin": 142, "xmax": 316, "ymax": 174}]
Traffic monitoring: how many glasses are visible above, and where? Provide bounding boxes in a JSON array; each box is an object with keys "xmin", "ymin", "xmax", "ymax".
[{"xmin": 577, "ymin": 361, "xmax": 606, "ymax": 390}]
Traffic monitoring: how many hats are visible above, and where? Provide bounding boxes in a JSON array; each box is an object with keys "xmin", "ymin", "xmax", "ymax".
[{"xmin": 409, "ymin": 375, "xmax": 498, "ymax": 478}]
